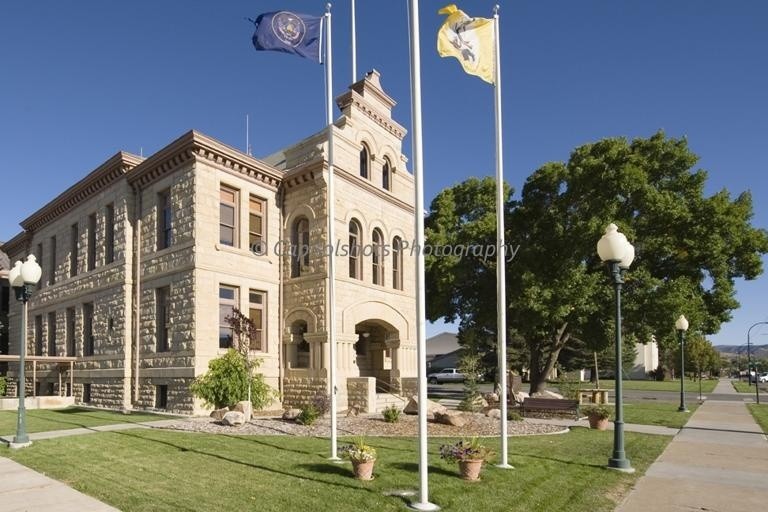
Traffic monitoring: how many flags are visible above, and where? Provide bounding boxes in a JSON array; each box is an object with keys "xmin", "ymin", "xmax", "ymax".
[
  {"xmin": 243, "ymin": 10, "xmax": 326, "ymax": 64},
  {"xmin": 437, "ymin": 4, "xmax": 496, "ymax": 86}
]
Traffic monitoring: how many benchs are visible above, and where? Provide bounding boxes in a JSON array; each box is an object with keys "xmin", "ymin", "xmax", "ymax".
[{"xmin": 522, "ymin": 397, "xmax": 580, "ymax": 422}]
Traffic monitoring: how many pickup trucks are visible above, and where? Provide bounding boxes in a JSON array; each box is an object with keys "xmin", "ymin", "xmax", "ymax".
[{"xmin": 427, "ymin": 365, "xmax": 488, "ymax": 385}]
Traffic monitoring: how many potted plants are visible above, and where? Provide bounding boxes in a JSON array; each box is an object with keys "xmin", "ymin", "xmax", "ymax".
[{"xmin": 581, "ymin": 405, "xmax": 611, "ymax": 430}]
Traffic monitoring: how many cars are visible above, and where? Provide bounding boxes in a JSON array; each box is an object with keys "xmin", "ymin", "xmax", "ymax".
[{"xmin": 738, "ymin": 368, "xmax": 768, "ymax": 384}]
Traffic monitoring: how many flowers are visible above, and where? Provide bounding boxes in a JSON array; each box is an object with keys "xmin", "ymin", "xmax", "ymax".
[
  {"xmin": 439, "ymin": 434, "xmax": 490, "ymax": 465},
  {"xmin": 337, "ymin": 431, "xmax": 376, "ymax": 461}
]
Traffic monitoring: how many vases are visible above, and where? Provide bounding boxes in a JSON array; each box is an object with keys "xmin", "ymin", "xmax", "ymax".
[
  {"xmin": 458, "ymin": 460, "xmax": 483, "ymax": 479},
  {"xmin": 351, "ymin": 459, "xmax": 376, "ymax": 480}
]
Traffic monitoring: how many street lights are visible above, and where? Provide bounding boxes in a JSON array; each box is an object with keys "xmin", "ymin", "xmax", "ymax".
[
  {"xmin": 5, "ymin": 251, "xmax": 44, "ymax": 449},
  {"xmin": 674, "ymin": 312, "xmax": 691, "ymax": 413},
  {"xmin": 595, "ymin": 220, "xmax": 638, "ymax": 469},
  {"xmin": 748, "ymin": 321, "xmax": 768, "ymax": 386}
]
